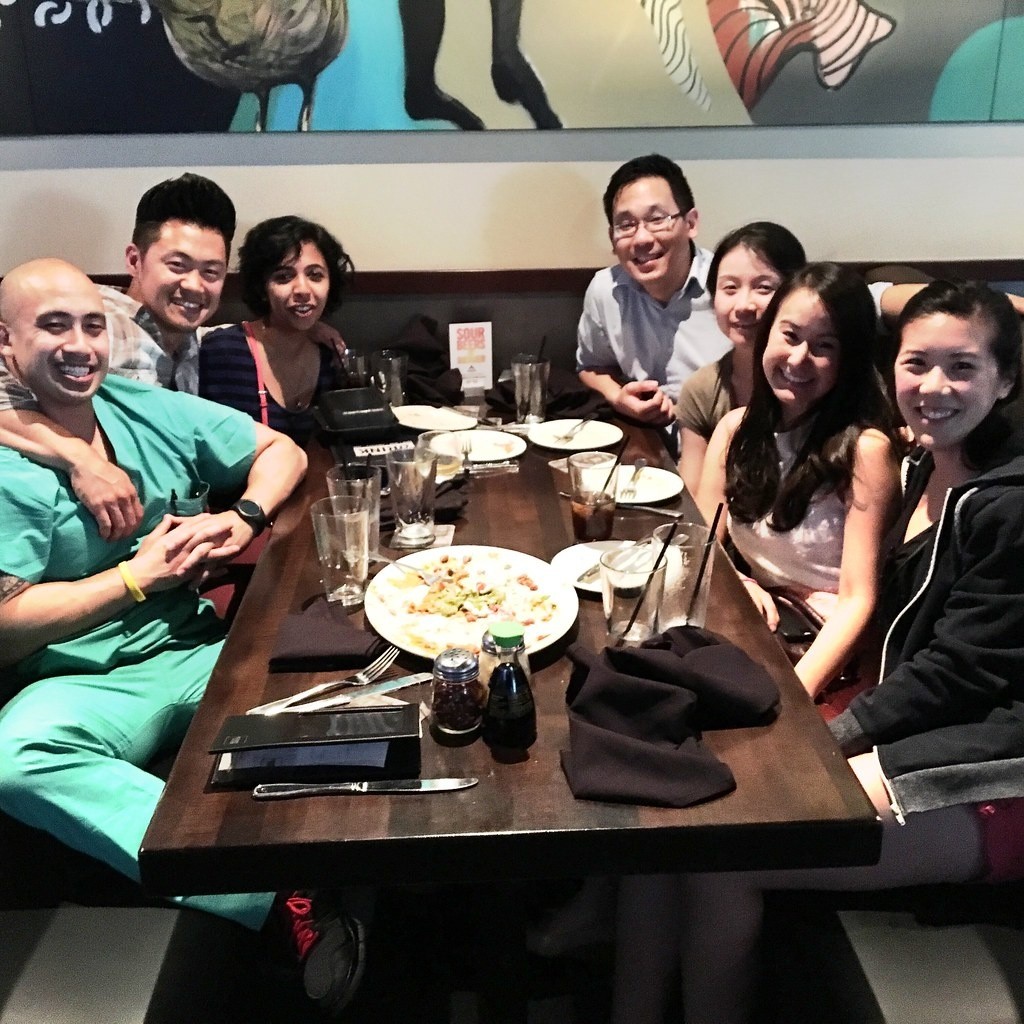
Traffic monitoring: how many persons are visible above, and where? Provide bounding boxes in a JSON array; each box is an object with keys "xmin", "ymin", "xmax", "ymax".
[
  {"xmin": 576, "ymin": 154, "xmax": 1024, "ymax": 428},
  {"xmin": 197, "ymin": 214, "xmax": 363, "ymax": 438},
  {"xmin": 0, "ymin": 256, "xmax": 365, "ymax": 1016},
  {"xmin": 0, "ymin": 174, "xmax": 349, "ymax": 542},
  {"xmin": 616, "ymin": 278, "xmax": 1024, "ymax": 1024},
  {"xmin": 678, "ymin": 222, "xmax": 898, "ymax": 501},
  {"xmin": 527, "ymin": 260, "xmax": 900, "ymax": 956}
]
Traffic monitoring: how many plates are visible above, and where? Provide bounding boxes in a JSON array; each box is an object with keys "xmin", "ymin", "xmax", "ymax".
[
  {"xmin": 431, "ymin": 430, "xmax": 527, "ymax": 463},
  {"xmin": 613, "ymin": 466, "xmax": 685, "ymax": 503},
  {"xmin": 364, "ymin": 544, "xmax": 579, "ymax": 658},
  {"xmin": 551, "ymin": 539, "xmax": 651, "ymax": 591},
  {"xmin": 527, "ymin": 418, "xmax": 622, "ymax": 450}
]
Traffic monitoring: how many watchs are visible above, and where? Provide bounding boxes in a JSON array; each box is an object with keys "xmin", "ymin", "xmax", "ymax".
[{"xmin": 230, "ymin": 498, "xmax": 266, "ymax": 537}]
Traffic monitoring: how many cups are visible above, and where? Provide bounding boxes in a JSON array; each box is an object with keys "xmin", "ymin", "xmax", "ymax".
[
  {"xmin": 567, "ymin": 451, "xmax": 619, "ymax": 545},
  {"xmin": 387, "ymin": 448, "xmax": 439, "ymax": 546},
  {"xmin": 341, "ymin": 346, "xmax": 368, "ymax": 380},
  {"xmin": 310, "ymin": 494, "xmax": 370, "ymax": 606},
  {"xmin": 512, "ymin": 354, "xmax": 550, "ymax": 423},
  {"xmin": 599, "ymin": 543, "xmax": 669, "ymax": 650},
  {"xmin": 369, "ymin": 348, "xmax": 408, "ymax": 406},
  {"xmin": 655, "ymin": 522, "xmax": 716, "ymax": 629},
  {"xmin": 324, "ymin": 464, "xmax": 381, "ymax": 565}
]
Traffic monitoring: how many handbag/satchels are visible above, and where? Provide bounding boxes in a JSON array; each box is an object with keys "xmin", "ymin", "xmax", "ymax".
[
  {"xmin": 196, "ymin": 491, "xmax": 271, "ymax": 621},
  {"xmin": 761, "ymin": 586, "xmax": 826, "ymax": 667}
]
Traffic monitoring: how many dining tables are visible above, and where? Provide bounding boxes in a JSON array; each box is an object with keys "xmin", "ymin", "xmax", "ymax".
[{"xmin": 137, "ymin": 355, "xmax": 883, "ymax": 1024}]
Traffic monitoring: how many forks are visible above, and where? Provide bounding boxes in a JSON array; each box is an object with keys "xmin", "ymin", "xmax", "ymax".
[
  {"xmin": 554, "ymin": 413, "xmax": 597, "ymax": 441},
  {"xmin": 460, "ymin": 439, "xmax": 472, "ymax": 468},
  {"xmin": 368, "ymin": 552, "xmax": 459, "ymax": 587},
  {"xmin": 244, "ymin": 646, "xmax": 400, "ymax": 715},
  {"xmin": 622, "ymin": 458, "xmax": 649, "ymax": 500}
]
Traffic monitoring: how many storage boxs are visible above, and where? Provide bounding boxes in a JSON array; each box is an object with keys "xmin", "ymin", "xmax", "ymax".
[{"xmin": 312, "ymin": 386, "xmax": 398, "ymax": 444}]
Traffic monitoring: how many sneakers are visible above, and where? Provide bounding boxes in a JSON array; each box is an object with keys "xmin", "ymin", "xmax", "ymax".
[{"xmin": 269, "ymin": 869, "xmax": 365, "ymax": 1019}]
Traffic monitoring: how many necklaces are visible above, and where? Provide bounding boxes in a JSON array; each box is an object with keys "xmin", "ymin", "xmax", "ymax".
[{"xmin": 260, "ymin": 320, "xmax": 317, "ymax": 411}]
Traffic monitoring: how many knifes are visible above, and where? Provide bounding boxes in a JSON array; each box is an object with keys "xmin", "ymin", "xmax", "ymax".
[
  {"xmin": 254, "ymin": 778, "xmax": 480, "ymax": 800},
  {"xmin": 577, "ymin": 521, "xmax": 666, "ymax": 583},
  {"xmin": 286, "ymin": 672, "xmax": 433, "ymax": 712},
  {"xmin": 559, "ymin": 491, "xmax": 685, "ymax": 519}
]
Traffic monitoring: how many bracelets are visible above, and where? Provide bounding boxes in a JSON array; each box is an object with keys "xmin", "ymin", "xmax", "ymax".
[{"xmin": 118, "ymin": 561, "xmax": 146, "ymax": 602}]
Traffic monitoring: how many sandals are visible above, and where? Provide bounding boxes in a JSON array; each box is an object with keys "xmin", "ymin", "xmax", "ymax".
[{"xmin": 525, "ymin": 911, "xmax": 613, "ymax": 955}]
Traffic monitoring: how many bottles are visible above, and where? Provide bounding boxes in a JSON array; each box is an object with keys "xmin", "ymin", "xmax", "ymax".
[{"xmin": 428, "ymin": 620, "xmax": 539, "ymax": 751}]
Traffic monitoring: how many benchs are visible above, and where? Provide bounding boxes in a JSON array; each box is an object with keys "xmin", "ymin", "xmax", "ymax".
[
  {"xmin": 0, "ymin": 904, "xmax": 226, "ymax": 1024},
  {"xmin": 835, "ymin": 903, "xmax": 1024, "ymax": 1024}
]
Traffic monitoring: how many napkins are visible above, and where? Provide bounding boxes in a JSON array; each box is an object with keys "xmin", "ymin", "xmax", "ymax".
[
  {"xmin": 269, "ymin": 590, "xmax": 388, "ymax": 673},
  {"xmin": 349, "ymin": 315, "xmax": 465, "ymax": 409},
  {"xmin": 378, "ymin": 468, "xmax": 473, "ymax": 531},
  {"xmin": 484, "ymin": 346, "xmax": 630, "ymax": 419},
  {"xmin": 559, "ymin": 627, "xmax": 782, "ymax": 811}
]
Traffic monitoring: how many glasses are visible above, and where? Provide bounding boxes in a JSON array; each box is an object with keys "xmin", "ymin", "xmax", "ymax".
[{"xmin": 609, "ymin": 211, "xmax": 683, "ymax": 236}]
